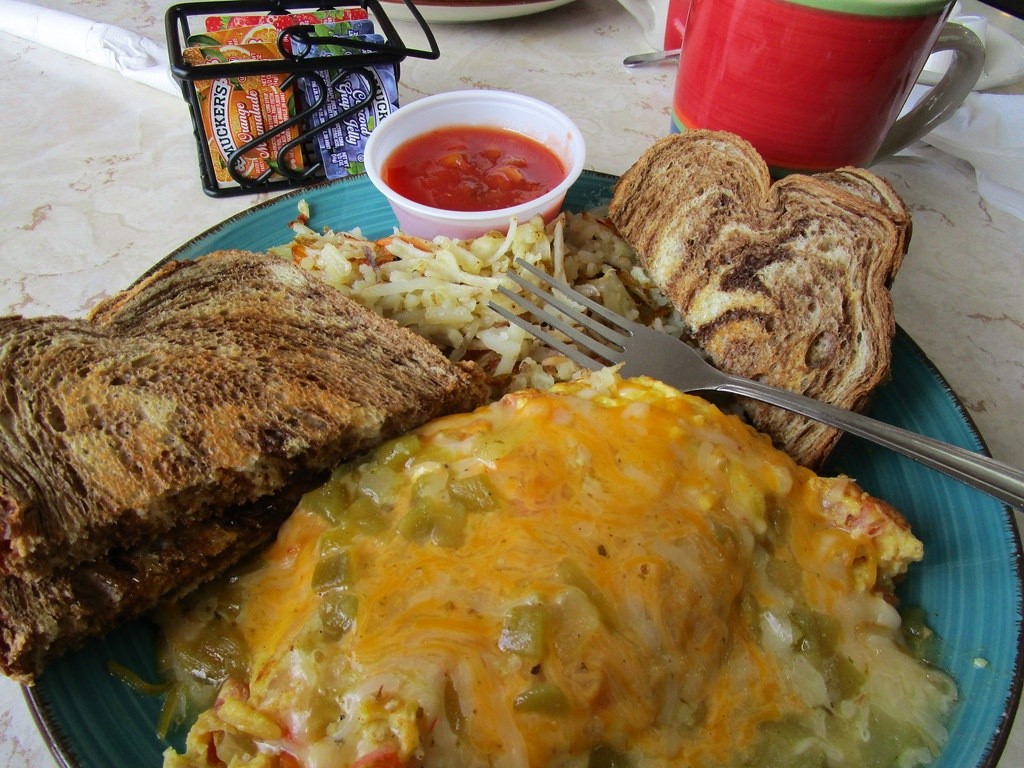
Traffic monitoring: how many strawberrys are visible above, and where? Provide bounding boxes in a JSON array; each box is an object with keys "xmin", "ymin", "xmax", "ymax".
[{"xmin": 205, "ymin": 8, "xmax": 368, "ymax": 54}]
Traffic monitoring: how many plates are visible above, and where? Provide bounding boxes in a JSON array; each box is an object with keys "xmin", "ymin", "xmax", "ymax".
[{"xmin": 27, "ymin": 169, "xmax": 1024, "ymax": 768}]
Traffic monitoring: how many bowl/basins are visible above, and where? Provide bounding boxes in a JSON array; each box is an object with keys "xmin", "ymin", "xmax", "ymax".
[{"xmin": 362, "ymin": 89, "xmax": 588, "ymax": 249}]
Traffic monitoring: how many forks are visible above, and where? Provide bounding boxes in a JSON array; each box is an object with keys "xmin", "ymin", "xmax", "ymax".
[{"xmin": 489, "ymin": 258, "xmax": 1024, "ymax": 515}]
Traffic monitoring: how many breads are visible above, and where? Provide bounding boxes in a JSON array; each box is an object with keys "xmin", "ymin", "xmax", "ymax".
[
  {"xmin": 0, "ymin": 249, "xmax": 498, "ymax": 680},
  {"xmin": 601, "ymin": 129, "xmax": 912, "ymax": 467}
]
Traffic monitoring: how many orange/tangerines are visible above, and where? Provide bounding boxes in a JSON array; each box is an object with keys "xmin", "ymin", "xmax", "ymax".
[{"xmin": 181, "ymin": 24, "xmax": 301, "ymax": 183}]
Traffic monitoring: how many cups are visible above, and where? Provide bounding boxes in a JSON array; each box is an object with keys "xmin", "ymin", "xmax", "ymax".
[{"xmin": 665, "ymin": 0, "xmax": 985, "ymax": 178}]
